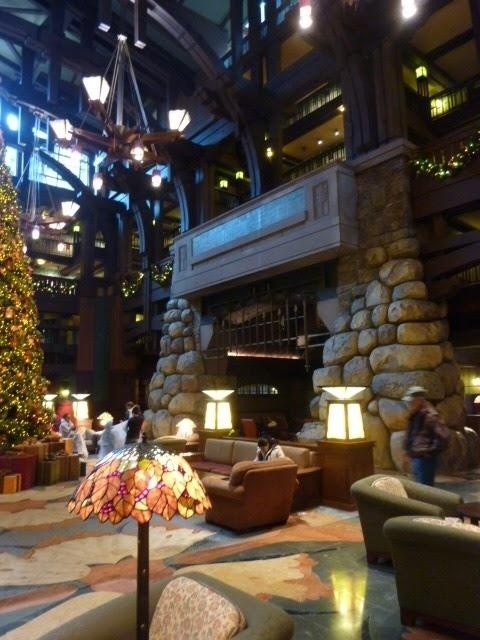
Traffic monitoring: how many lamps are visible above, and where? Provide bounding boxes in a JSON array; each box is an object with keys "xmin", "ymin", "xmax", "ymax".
[
  {"xmin": 234, "ymin": 166, "xmax": 246, "ymax": 184},
  {"xmin": 40, "ymin": 389, "xmax": 58, "ymax": 410},
  {"xmin": 11, "ymin": 151, "xmax": 82, "ymax": 258},
  {"xmin": 413, "ymin": 63, "xmax": 435, "ymax": 98},
  {"xmin": 41, "ymin": 34, "xmax": 194, "ymax": 192},
  {"xmin": 217, "ymin": 174, "xmax": 230, "ymax": 193},
  {"xmin": 69, "ymin": 390, "xmax": 91, "ymax": 423},
  {"xmin": 321, "ymin": 382, "xmax": 374, "ymax": 440},
  {"xmin": 62, "ymin": 432, "xmax": 213, "ymax": 640},
  {"xmin": 197, "ymin": 386, "xmax": 235, "ymax": 428}
]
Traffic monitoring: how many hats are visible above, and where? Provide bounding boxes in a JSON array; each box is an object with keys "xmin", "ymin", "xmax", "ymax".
[{"xmin": 406, "ymin": 386, "xmax": 428, "ymax": 395}]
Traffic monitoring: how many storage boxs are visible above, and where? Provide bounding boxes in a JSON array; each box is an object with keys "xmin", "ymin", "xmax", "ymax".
[{"xmin": 0, "ymin": 433, "xmax": 85, "ymax": 500}]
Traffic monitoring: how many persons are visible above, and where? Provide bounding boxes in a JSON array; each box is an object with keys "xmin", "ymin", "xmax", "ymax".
[
  {"xmin": 402, "ymin": 386, "xmax": 450, "ymax": 487},
  {"xmin": 253, "ymin": 436, "xmax": 285, "ymax": 462},
  {"xmin": 50, "ymin": 401, "xmax": 145, "ymax": 460}
]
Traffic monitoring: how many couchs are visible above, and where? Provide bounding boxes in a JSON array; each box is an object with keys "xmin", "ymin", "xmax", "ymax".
[
  {"xmin": 197, "ymin": 453, "xmax": 302, "ymax": 533},
  {"xmin": 177, "ymin": 433, "xmax": 323, "ymax": 515},
  {"xmin": 350, "ymin": 471, "xmax": 466, "ymax": 567},
  {"xmin": 28, "ymin": 568, "xmax": 300, "ymax": 640},
  {"xmin": 379, "ymin": 509, "xmax": 480, "ymax": 638}
]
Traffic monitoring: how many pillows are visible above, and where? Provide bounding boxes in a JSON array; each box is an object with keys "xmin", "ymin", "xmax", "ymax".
[{"xmin": 144, "ymin": 575, "xmax": 246, "ymax": 640}]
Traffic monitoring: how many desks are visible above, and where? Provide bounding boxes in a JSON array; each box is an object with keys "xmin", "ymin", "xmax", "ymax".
[{"xmin": 446, "ymin": 502, "xmax": 480, "ymax": 522}]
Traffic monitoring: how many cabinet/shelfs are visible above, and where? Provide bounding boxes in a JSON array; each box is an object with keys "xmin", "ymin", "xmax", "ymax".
[
  {"xmin": 316, "ymin": 437, "xmax": 376, "ymax": 511},
  {"xmin": 192, "ymin": 429, "xmax": 234, "ymax": 452}
]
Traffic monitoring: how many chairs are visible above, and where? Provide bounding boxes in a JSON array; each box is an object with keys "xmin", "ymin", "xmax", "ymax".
[{"xmin": 153, "ymin": 432, "xmax": 199, "ymax": 453}]
